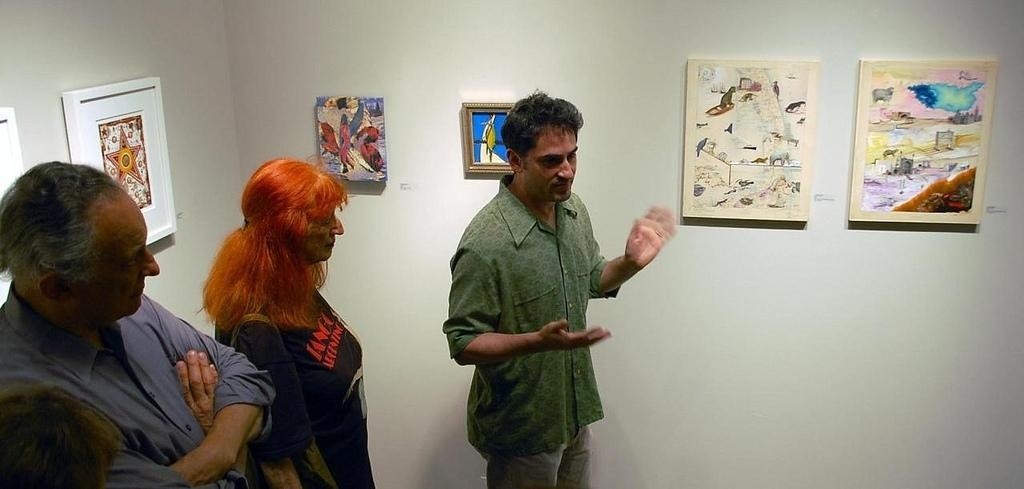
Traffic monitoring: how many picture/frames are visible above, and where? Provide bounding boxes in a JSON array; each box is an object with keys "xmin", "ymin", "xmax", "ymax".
[
  {"xmin": 678, "ymin": 56, "xmax": 820, "ymax": 227},
  {"xmin": 0, "ymin": 102, "xmax": 25, "ymax": 311},
  {"xmin": 461, "ymin": 101, "xmax": 516, "ymax": 175},
  {"xmin": 60, "ymin": 71, "xmax": 180, "ymax": 246},
  {"xmin": 846, "ymin": 55, "xmax": 1001, "ymax": 231}
]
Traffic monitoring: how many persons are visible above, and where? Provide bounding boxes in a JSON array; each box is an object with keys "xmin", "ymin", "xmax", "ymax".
[
  {"xmin": 202, "ymin": 159, "xmax": 375, "ymax": 489},
  {"xmin": 442, "ymin": 92, "xmax": 678, "ymax": 489},
  {"xmin": 0, "ymin": 384, "xmax": 121, "ymax": 489},
  {"xmin": 0, "ymin": 162, "xmax": 276, "ymax": 489}
]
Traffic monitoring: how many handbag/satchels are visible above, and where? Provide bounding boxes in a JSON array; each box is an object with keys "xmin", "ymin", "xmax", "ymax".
[{"xmin": 229, "ymin": 312, "xmax": 341, "ymax": 489}]
